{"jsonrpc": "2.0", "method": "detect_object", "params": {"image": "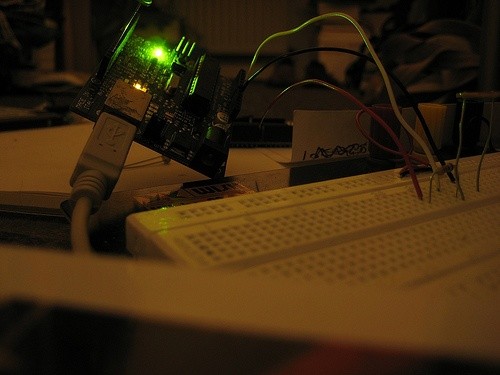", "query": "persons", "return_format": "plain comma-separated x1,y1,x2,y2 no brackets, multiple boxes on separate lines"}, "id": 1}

0,0,92,87
